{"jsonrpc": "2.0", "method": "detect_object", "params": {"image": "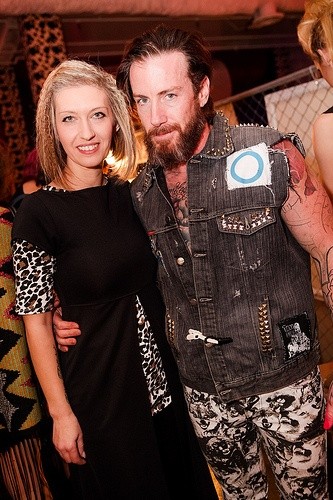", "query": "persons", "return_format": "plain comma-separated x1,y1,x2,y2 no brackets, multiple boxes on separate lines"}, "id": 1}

9,146,48,221
296,0,333,202
1,193,60,500
12,58,220,499
50,22,333,499
0,141,17,210
